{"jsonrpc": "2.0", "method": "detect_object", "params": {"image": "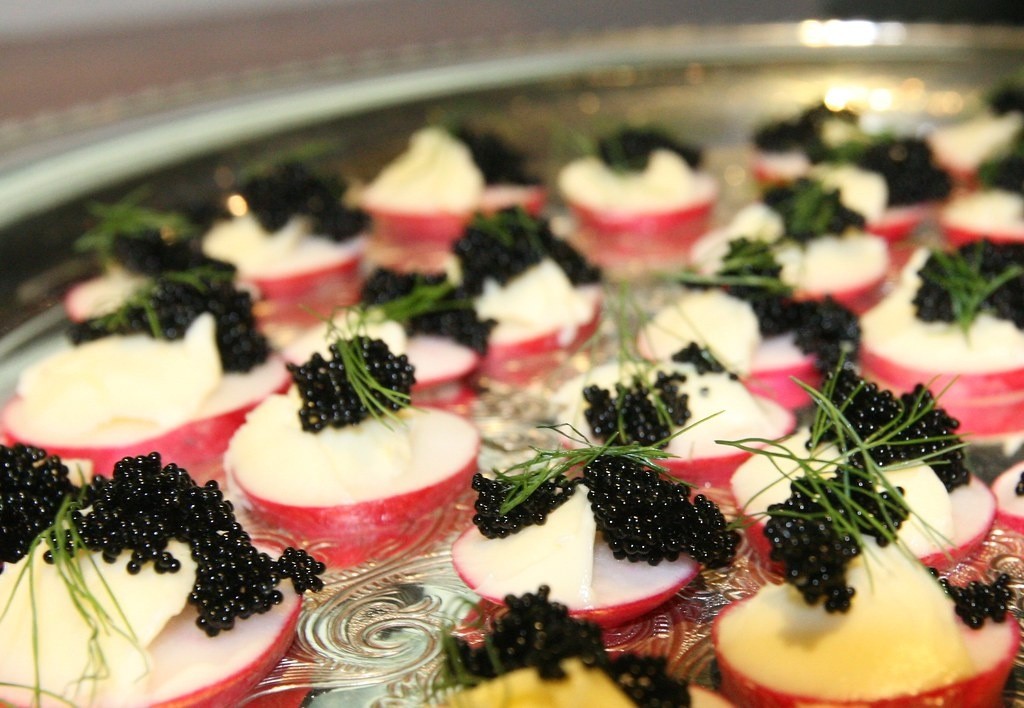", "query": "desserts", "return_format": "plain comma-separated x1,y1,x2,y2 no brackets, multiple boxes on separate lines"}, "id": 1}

1,83,1024,708
